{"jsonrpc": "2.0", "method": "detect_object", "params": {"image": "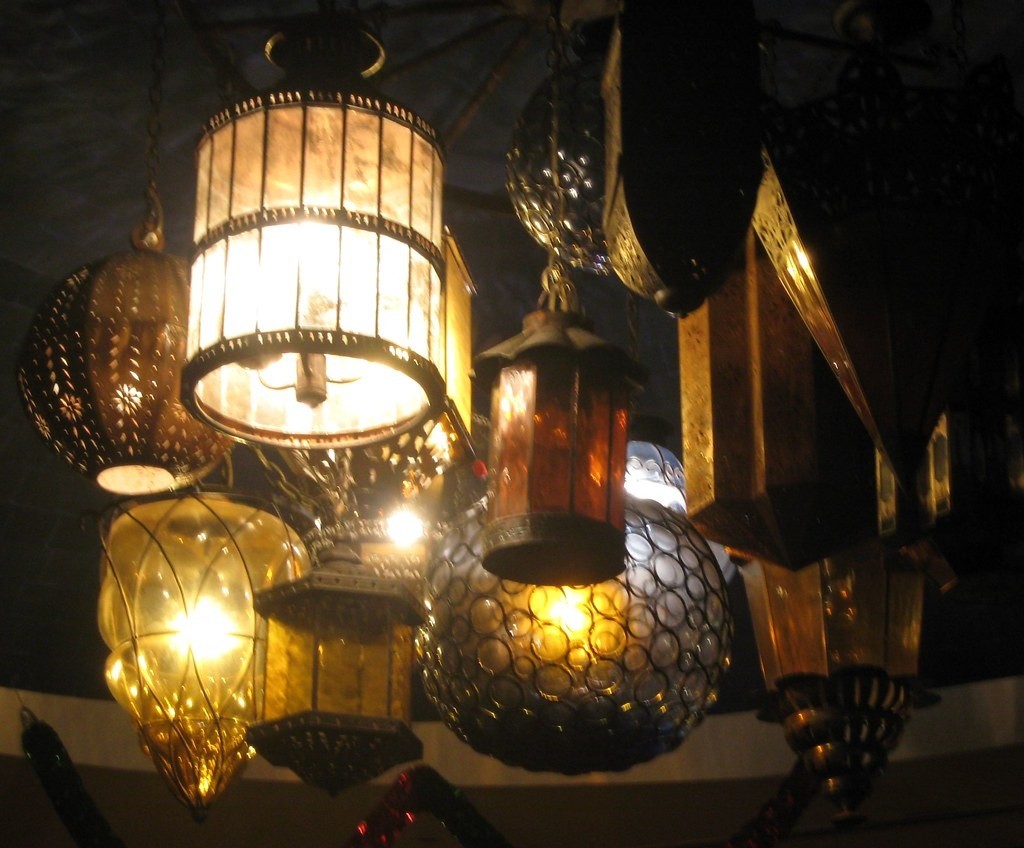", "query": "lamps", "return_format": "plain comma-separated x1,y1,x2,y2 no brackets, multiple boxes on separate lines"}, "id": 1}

0,0,1024,848
